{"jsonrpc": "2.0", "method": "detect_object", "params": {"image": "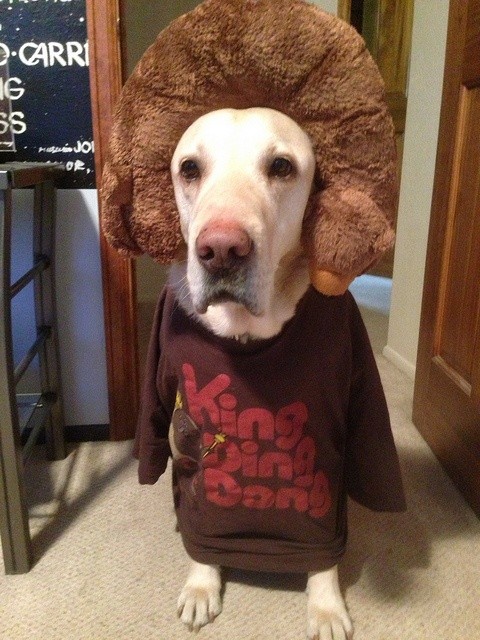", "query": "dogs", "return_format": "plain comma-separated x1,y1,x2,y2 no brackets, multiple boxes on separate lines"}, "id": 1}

168,106,354,640
